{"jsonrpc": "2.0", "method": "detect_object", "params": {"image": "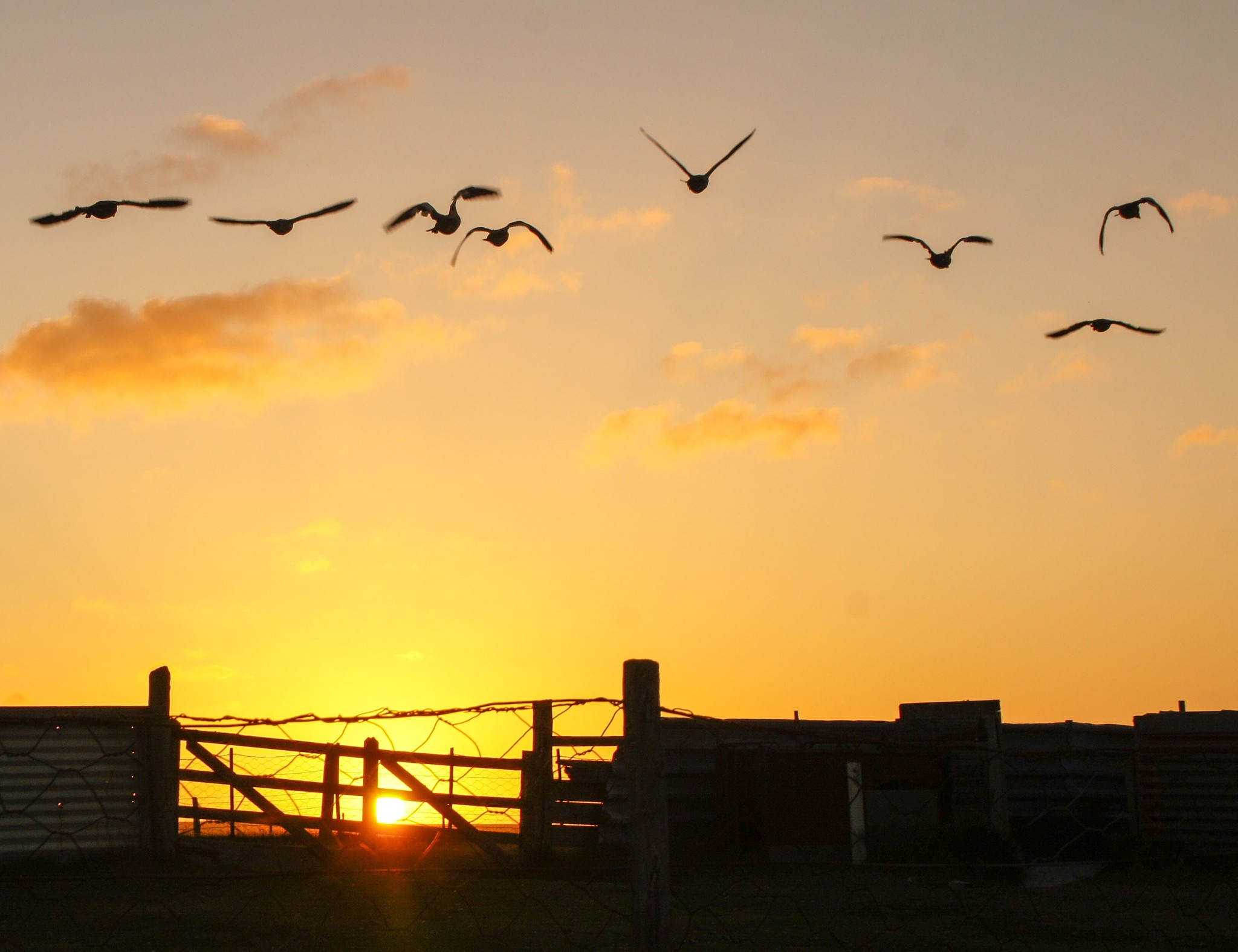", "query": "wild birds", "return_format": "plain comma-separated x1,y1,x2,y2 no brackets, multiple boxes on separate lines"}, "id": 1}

1044,319,1167,340
637,125,758,193
26,185,554,267
1099,197,1174,256
879,232,995,269
